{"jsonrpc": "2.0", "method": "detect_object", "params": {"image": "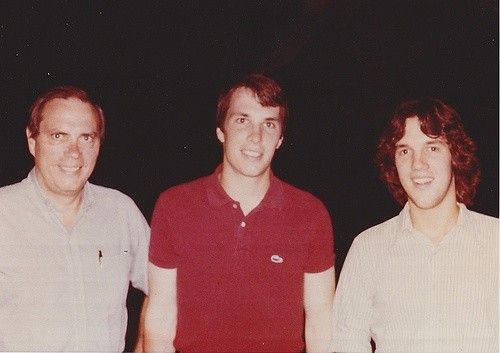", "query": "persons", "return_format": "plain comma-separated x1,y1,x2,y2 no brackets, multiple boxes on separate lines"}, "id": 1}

146,74,336,353
331,97,500,353
0,86,151,353
